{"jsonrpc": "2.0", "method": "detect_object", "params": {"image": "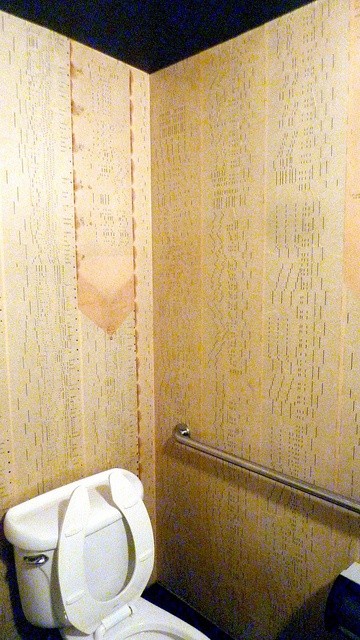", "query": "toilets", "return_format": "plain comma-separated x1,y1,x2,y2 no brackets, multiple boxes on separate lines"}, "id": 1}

3,466,215,640
